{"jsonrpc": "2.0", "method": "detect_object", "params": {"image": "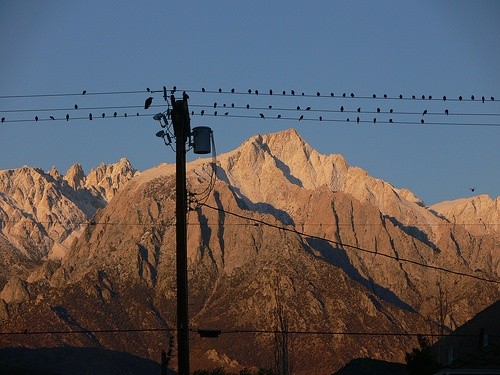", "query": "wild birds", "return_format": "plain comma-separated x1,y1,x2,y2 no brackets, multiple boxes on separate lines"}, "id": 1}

246,103,250,110
213,102,235,116
231,88,235,94
259,105,282,119
442,95,447,102
1,117,6,124
34,103,78,122
201,87,206,93
339,105,361,124
420,109,428,124
445,109,449,116
218,87,222,93
469,186,477,192
269,88,433,101
136,112,140,116
191,108,205,116
373,107,394,124
173,85,177,90
248,88,259,95
182,94,190,101
82,90,87,95
458,94,495,104
144,87,154,110
296,105,323,122
88,111,128,121
